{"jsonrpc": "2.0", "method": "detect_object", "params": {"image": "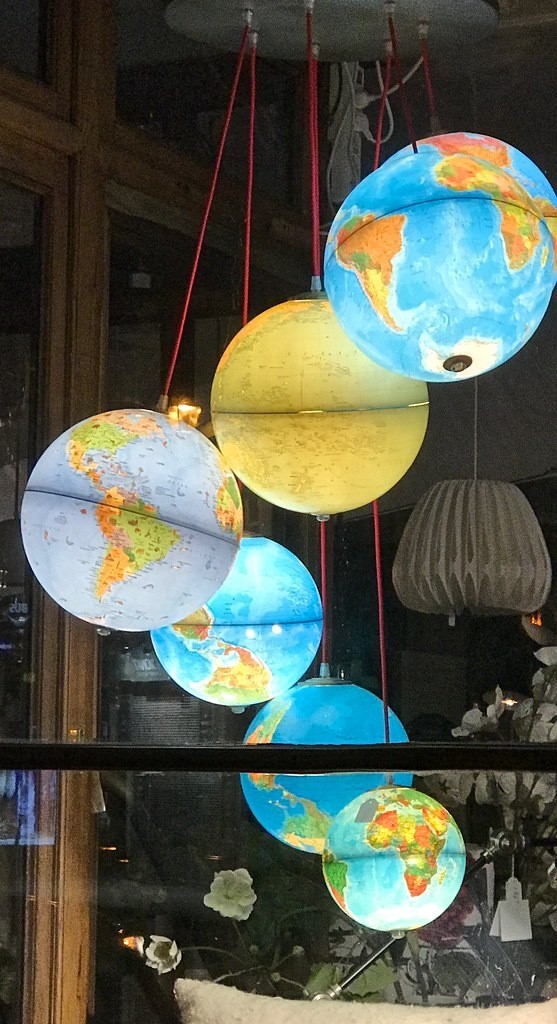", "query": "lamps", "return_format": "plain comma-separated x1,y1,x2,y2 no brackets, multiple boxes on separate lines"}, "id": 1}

209,3,428,513
320,499,467,932
19,7,252,636
150,34,322,706
240,523,412,853
323,2,557,383
391,376,552,617
